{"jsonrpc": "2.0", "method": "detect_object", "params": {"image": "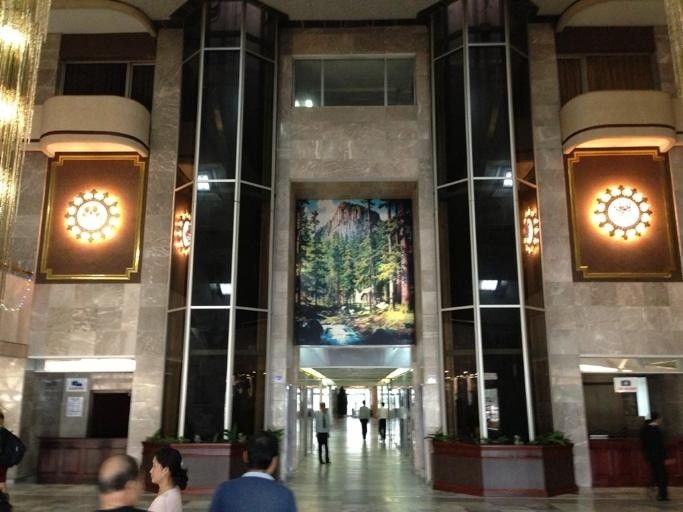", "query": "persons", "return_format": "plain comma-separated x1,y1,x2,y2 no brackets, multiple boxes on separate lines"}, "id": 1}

374,402,386,441
86,453,153,511
0,412,25,492
146,446,188,511
357,400,370,439
315,402,332,463
640,411,677,501
207,430,296,511
